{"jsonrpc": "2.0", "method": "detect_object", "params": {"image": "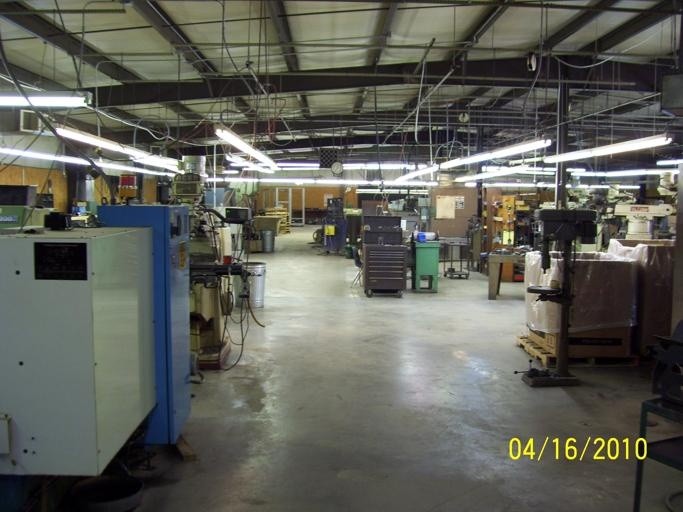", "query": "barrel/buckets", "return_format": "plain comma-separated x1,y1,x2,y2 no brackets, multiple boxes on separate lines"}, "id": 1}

234,262,267,309
260,229,275,252
